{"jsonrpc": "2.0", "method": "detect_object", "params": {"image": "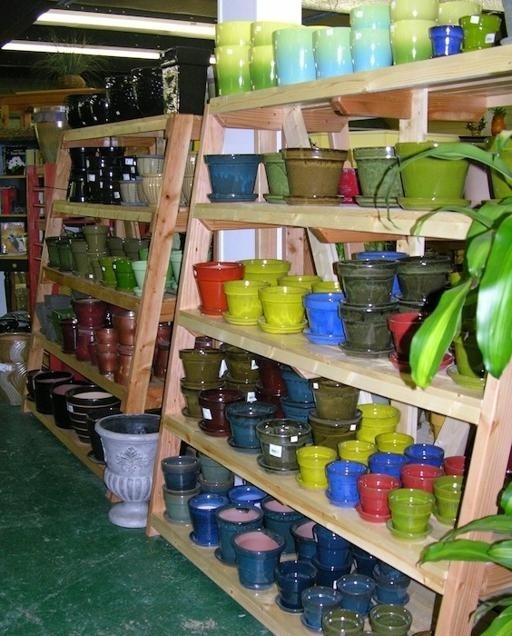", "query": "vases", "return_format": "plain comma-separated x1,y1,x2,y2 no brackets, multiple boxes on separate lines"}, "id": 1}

94,413,163,529
158,2,508,636
3,45,208,463
